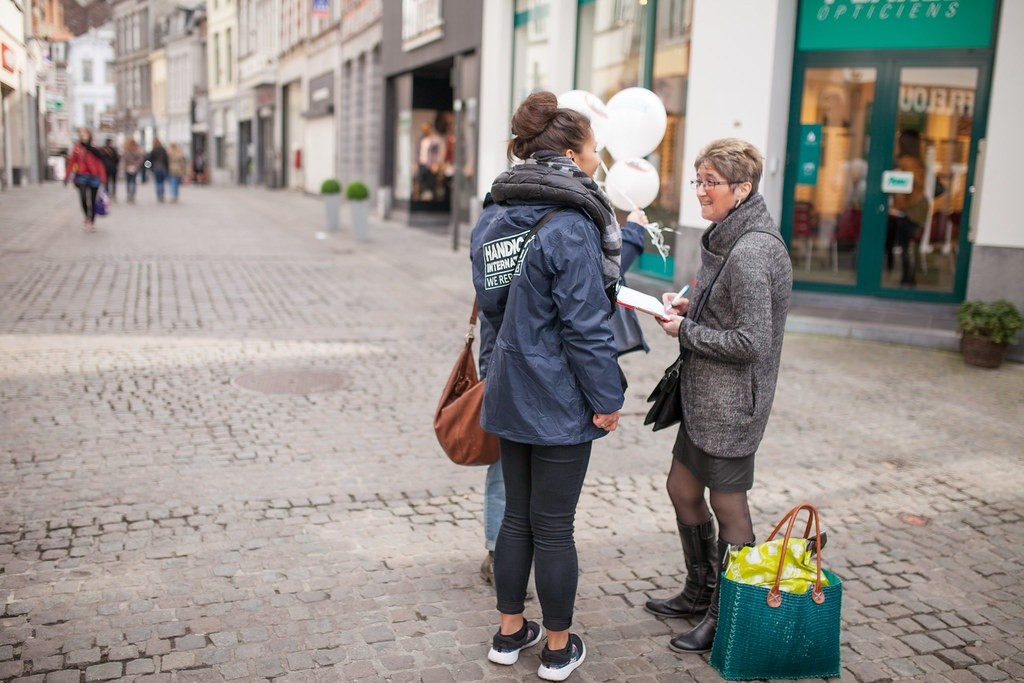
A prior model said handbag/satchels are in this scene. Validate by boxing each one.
[607,277,650,357]
[95,184,110,214]
[706,504,843,680]
[644,354,683,431]
[433,338,500,466]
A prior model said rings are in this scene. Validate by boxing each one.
[601,424,607,429]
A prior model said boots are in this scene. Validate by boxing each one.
[643,513,718,617]
[668,531,756,654]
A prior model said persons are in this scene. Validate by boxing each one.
[99,138,120,201]
[147,136,169,203]
[417,119,455,211]
[139,148,149,182]
[883,130,948,289]
[64,127,107,232]
[125,138,146,203]
[166,142,187,203]
[644,139,793,655]
[470,91,648,681]
[194,147,205,182]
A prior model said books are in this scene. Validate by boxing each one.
[615,285,670,322]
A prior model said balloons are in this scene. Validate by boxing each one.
[605,87,667,161]
[605,158,660,212]
[557,90,610,153]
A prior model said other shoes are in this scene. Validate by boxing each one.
[480,554,535,599]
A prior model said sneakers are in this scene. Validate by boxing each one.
[537,632,586,681]
[487,617,542,665]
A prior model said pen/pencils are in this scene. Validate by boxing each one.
[663,284,691,312]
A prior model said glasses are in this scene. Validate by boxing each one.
[691,179,744,190]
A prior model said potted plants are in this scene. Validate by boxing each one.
[306,179,340,228]
[957,300,1024,368]
[345,183,370,240]
